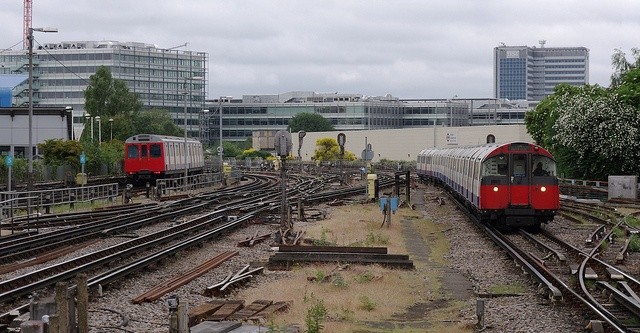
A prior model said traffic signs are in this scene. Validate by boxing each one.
[6,156,13,166]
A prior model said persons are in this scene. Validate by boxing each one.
[534,162,551,177]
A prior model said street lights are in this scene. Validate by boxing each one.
[85,114,93,154]
[65,106,73,140]
[184,77,202,184]
[28,27,58,182]
[219,96,233,171]
[95,116,101,156]
[199,110,209,142]
[109,118,114,140]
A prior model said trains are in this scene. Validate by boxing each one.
[123,134,204,186]
[417,142,559,225]
